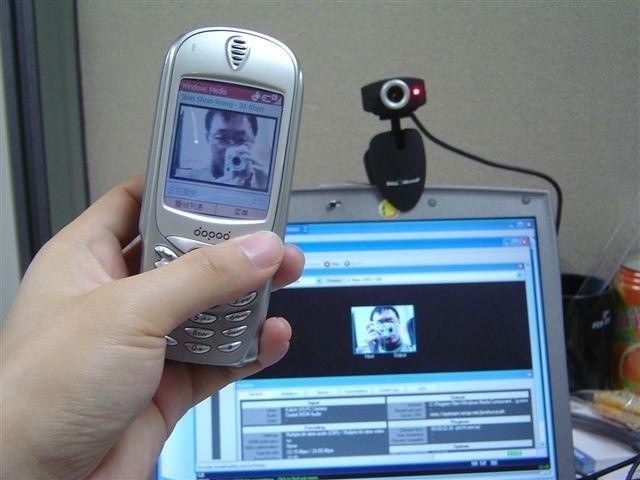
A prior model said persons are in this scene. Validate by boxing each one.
[0,174,307,480]
[356,307,411,352]
[189,109,269,192]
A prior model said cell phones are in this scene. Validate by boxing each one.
[136,28,303,369]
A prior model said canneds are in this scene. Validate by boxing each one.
[611,259,640,396]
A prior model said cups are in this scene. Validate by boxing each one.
[561,272,613,395]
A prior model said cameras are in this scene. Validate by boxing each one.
[222,146,250,187]
[375,323,395,335]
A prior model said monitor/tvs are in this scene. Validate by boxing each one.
[155,184,577,480]
